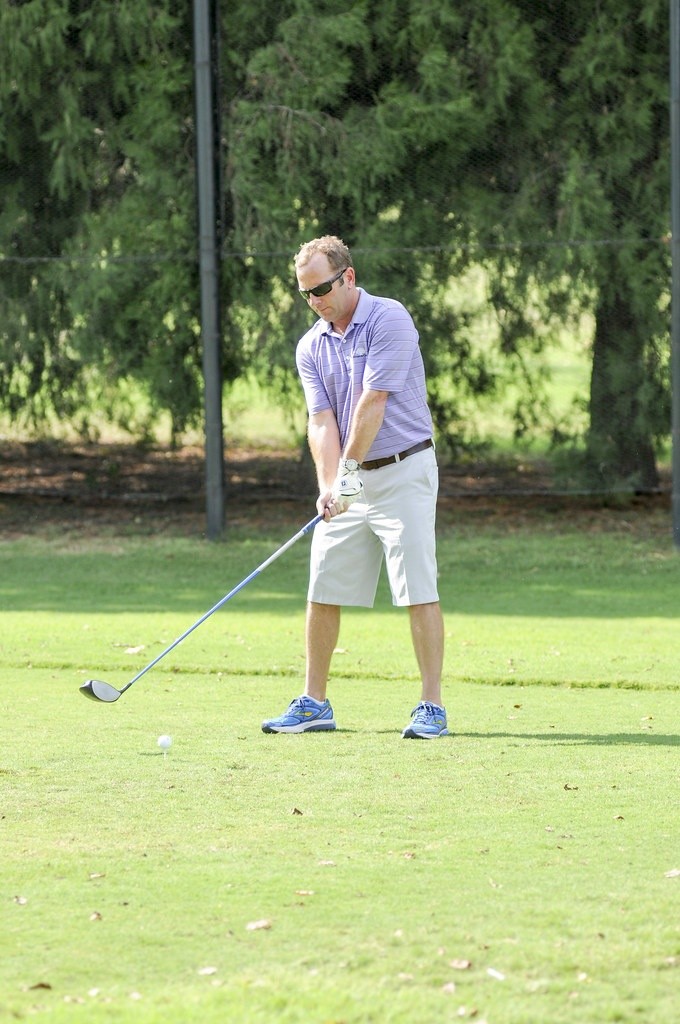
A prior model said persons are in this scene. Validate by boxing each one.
[261,235,448,739]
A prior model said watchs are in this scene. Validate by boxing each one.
[340,459,362,471]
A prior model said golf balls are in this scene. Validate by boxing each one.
[157,734,173,750]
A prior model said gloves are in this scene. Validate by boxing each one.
[331,458,363,512]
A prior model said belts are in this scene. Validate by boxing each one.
[360,438,433,470]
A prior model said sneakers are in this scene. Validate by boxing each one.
[402,701,448,739]
[261,696,336,734]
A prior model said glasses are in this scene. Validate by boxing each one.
[299,269,346,299]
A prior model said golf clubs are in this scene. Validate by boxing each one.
[78,511,326,704]
